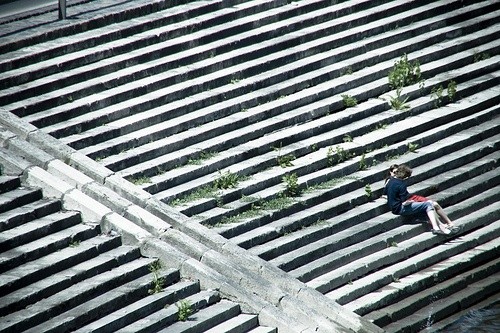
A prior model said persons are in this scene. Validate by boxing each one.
[382,164,460,234]
[387,165,451,235]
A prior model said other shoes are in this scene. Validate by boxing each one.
[432,229,444,234]
